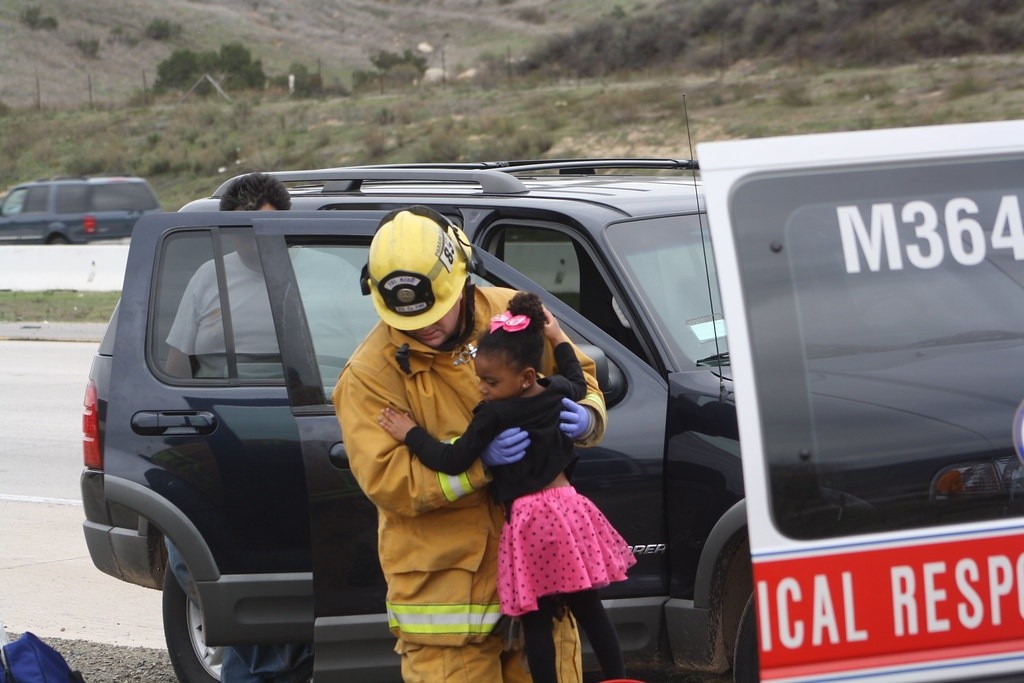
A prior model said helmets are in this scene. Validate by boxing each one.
[361,208,472,330]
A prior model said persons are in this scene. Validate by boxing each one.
[163,173,386,683]
[380,293,637,683]
[332,205,606,683]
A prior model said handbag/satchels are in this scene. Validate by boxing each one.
[0,630,84,683]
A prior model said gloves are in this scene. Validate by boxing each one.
[558,397,590,438]
[479,427,531,468]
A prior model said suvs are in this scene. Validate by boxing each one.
[0,176,160,245]
[77,158,1024,683]
